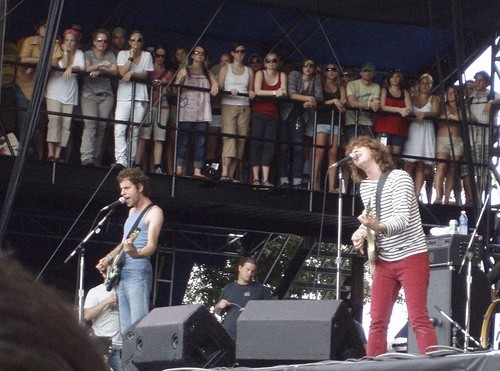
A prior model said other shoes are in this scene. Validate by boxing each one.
[82,159,95,165]
[252,180,275,187]
[192,174,208,179]
[47,156,64,163]
[220,176,241,183]
[279,184,303,190]
[464,202,473,207]
[434,199,442,204]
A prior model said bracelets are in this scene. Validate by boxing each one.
[273,90,276,96]
[366,101,370,108]
[128,57,134,62]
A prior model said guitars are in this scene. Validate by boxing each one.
[364,199,378,276]
[104,227,141,291]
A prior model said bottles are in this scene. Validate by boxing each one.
[459,211,468,235]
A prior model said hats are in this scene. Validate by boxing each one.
[419,70,433,81]
[361,62,375,72]
[64,29,80,40]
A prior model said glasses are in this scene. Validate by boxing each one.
[40,25,46,28]
[73,28,83,33]
[130,38,142,42]
[265,59,278,63]
[326,68,337,72]
[155,53,166,58]
[194,51,206,56]
[97,38,108,44]
[235,50,245,54]
[304,63,315,68]
[390,68,400,72]
[342,72,354,77]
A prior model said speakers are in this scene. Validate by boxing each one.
[236,299,367,367]
[121,314,146,371]
[134,303,236,371]
[406,265,491,355]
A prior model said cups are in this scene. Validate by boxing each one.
[449,220,458,234]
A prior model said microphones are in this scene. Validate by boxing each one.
[100,197,126,212]
[429,317,443,326]
[330,152,357,168]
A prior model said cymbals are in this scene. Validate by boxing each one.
[487,252,500,257]
[486,243,500,248]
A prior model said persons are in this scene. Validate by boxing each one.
[214,256,270,342]
[0,19,500,208]
[83,264,124,371]
[344,136,438,356]
[0,253,109,371]
[95,167,165,334]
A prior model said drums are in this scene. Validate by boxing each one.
[479,298,500,350]
